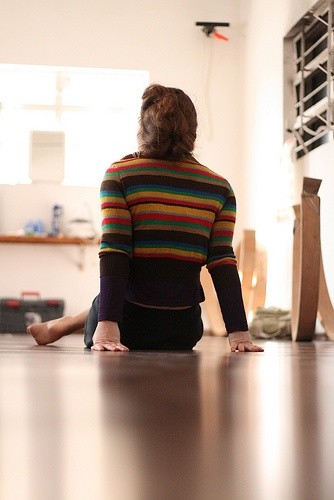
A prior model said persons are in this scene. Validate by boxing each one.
[26,84,263,353]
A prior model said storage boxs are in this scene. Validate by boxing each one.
[0,291,65,335]
